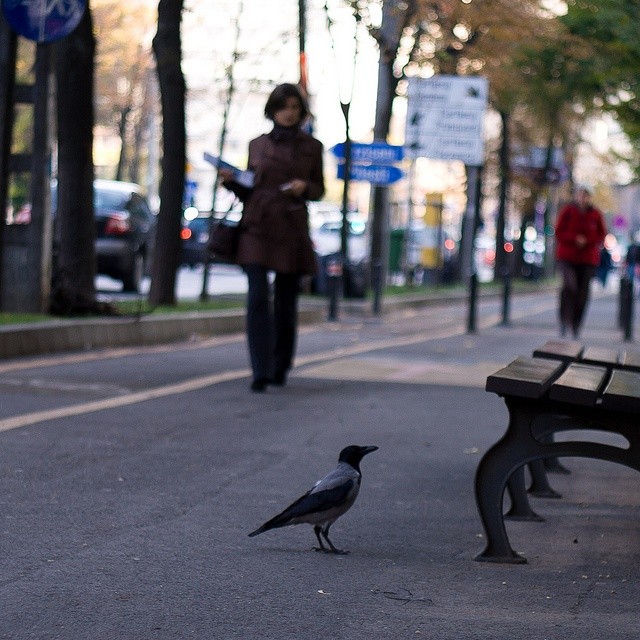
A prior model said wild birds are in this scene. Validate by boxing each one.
[248,445,380,555]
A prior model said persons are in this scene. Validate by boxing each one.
[204,84,327,394]
[550,184,607,343]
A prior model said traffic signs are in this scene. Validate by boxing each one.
[337,163,403,186]
[328,141,404,165]
[406,78,487,166]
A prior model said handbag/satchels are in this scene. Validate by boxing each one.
[207,192,246,257]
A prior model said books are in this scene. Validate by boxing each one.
[205,153,255,190]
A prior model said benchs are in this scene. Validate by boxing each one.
[470,336,638,564]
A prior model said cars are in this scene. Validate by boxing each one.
[48,178,157,295]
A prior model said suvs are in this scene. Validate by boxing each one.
[310,216,370,299]
[182,216,247,274]
